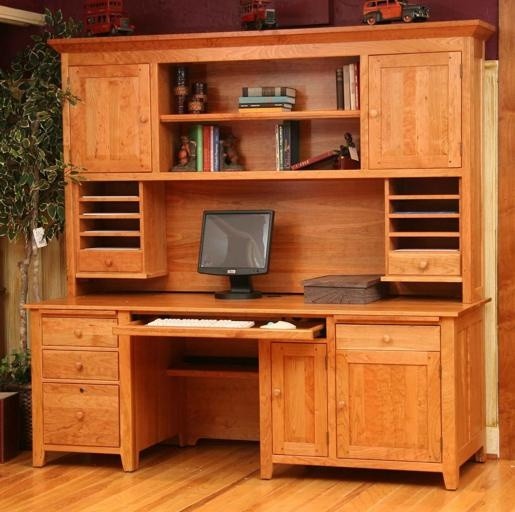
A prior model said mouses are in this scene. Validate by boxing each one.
[260,319,297,330]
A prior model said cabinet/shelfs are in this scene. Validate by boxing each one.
[42,35,483,306]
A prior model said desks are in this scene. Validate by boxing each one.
[20,293,490,488]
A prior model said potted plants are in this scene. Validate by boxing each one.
[0,6,85,452]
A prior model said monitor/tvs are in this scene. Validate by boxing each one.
[196,209,276,300]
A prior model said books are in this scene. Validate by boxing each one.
[336,62,360,111]
[274,119,341,171]
[238,86,296,114]
[196,124,220,171]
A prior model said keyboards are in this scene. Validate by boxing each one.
[147,317,255,329]
